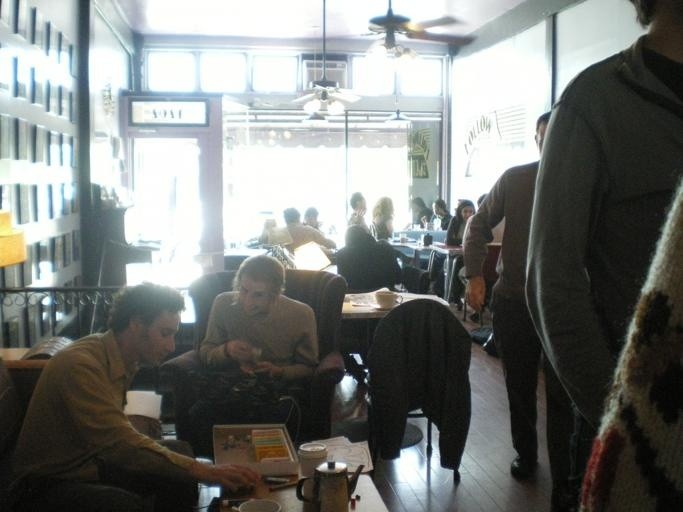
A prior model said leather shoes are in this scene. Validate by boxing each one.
[511,455,538,479]
[483,333,497,355]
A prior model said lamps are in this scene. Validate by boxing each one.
[301,96,347,117]
[384,67,413,128]
[367,39,420,61]
[0,211,27,289]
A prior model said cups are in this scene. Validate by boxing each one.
[296,441,328,477]
[234,497,281,512]
[374,289,405,308]
[398,232,408,247]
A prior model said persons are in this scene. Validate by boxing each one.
[0,280,259,511]
[251,188,506,357]
[524,0,683,512]
[174,255,320,456]
[462,109,574,481]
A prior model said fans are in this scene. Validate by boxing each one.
[292,1,360,118]
[360,0,475,62]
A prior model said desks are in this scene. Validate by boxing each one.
[197,435,391,512]
[180,293,448,329]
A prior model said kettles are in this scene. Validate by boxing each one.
[295,457,366,511]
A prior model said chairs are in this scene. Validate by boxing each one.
[0,357,196,511]
[347,230,500,485]
[187,267,349,444]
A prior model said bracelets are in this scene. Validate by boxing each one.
[223,341,232,360]
